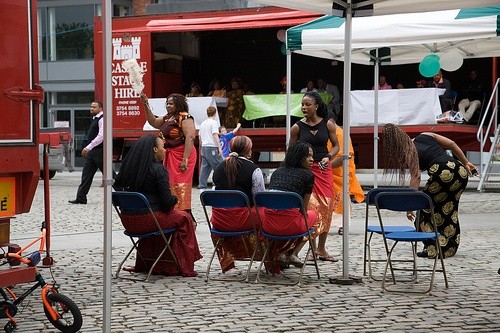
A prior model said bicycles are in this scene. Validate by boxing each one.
[0,220,83,333]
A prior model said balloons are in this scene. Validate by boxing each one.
[419,49,463,79]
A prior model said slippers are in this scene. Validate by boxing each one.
[319,255,338,262]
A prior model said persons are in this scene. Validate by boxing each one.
[395,82,404,89]
[300,81,318,94]
[317,77,342,122]
[267,141,321,268]
[69,102,118,204]
[426,71,452,114]
[370,74,394,90]
[187,83,202,98]
[381,123,480,259]
[416,79,427,87]
[223,78,245,128]
[198,108,242,188]
[278,74,293,94]
[287,92,365,261]
[207,81,227,98]
[213,136,266,274]
[139,92,197,229]
[112,134,202,278]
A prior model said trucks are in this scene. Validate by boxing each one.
[0,1,71,262]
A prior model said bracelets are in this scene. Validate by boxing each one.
[182,158,189,161]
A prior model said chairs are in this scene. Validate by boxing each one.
[362,187,420,282]
[111,191,181,283]
[200,190,271,283]
[253,192,321,287]
[451,90,486,126]
[375,191,449,297]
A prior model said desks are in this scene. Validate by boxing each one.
[143,96,228,132]
[349,88,447,127]
[241,91,334,128]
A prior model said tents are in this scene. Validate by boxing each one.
[246,0,500,284]
[286,5,500,161]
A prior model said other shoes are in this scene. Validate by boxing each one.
[68,199,87,204]
[290,256,304,267]
